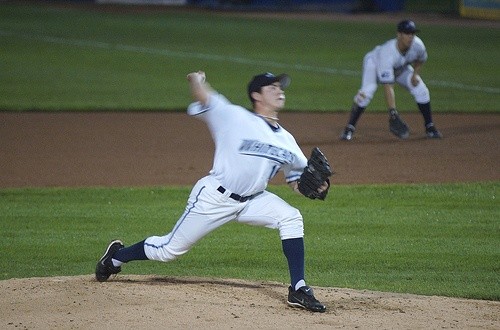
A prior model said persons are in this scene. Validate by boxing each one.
[96,70,331,311]
[339,20,444,140]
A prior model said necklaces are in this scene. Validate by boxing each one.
[256,113,279,121]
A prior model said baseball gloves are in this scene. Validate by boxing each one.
[388,108,409,141]
[298,147,335,201]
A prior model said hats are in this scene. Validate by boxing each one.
[248,73,291,101]
[398,20,421,34]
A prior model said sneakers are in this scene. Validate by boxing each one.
[96,239,124,282]
[425,126,444,139]
[288,284,326,313]
[341,128,353,143]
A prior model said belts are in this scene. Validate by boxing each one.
[217,186,264,202]
